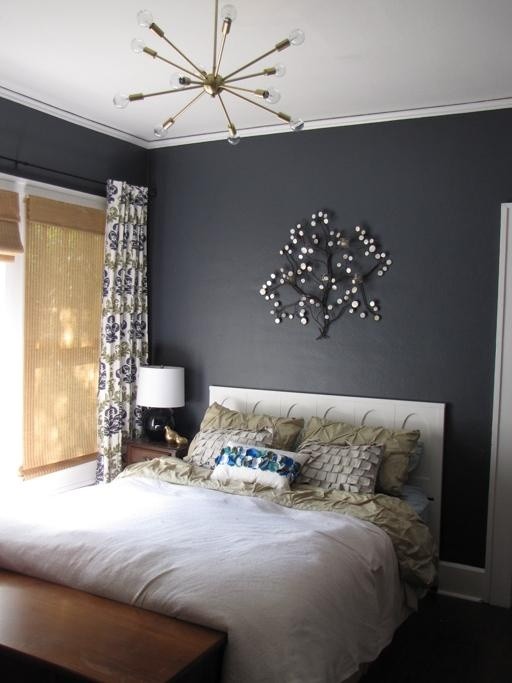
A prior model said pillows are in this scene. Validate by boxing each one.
[183,401,425,495]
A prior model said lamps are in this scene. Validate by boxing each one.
[112,1,306,145]
[135,363,187,442]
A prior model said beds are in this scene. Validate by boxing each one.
[0,384,446,682]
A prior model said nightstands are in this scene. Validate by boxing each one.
[126,439,189,467]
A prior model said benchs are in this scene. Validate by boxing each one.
[0,570,229,680]
